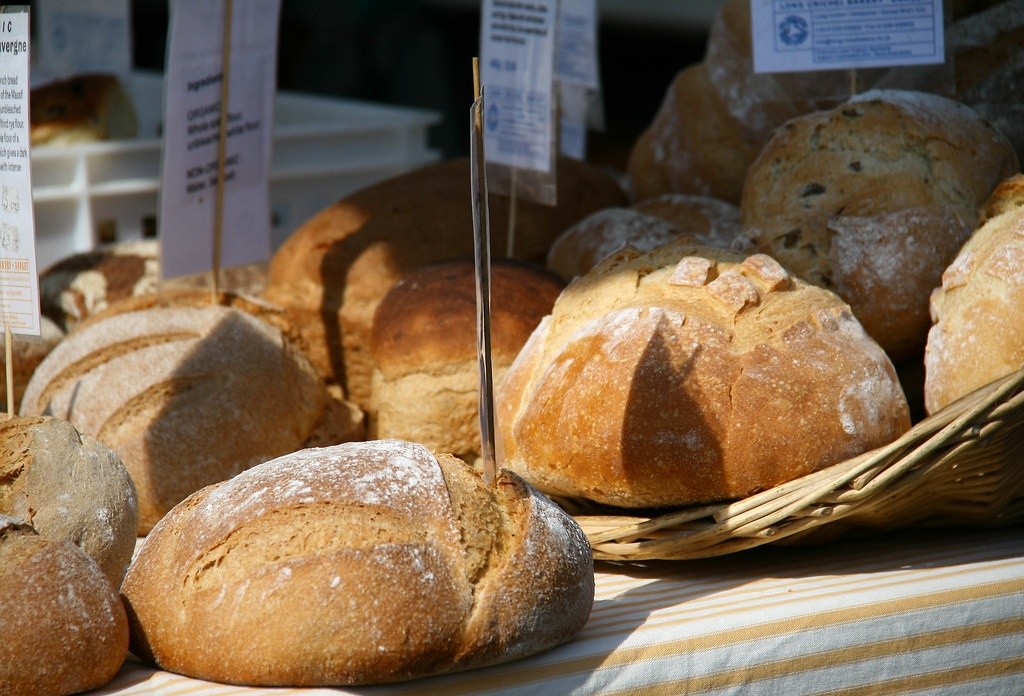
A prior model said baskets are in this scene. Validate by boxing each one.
[461,365,1024,564]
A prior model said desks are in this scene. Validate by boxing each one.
[83,516,1024,696]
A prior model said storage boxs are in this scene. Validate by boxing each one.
[29,77,433,274]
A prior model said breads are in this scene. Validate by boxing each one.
[0,0,1024,696]
